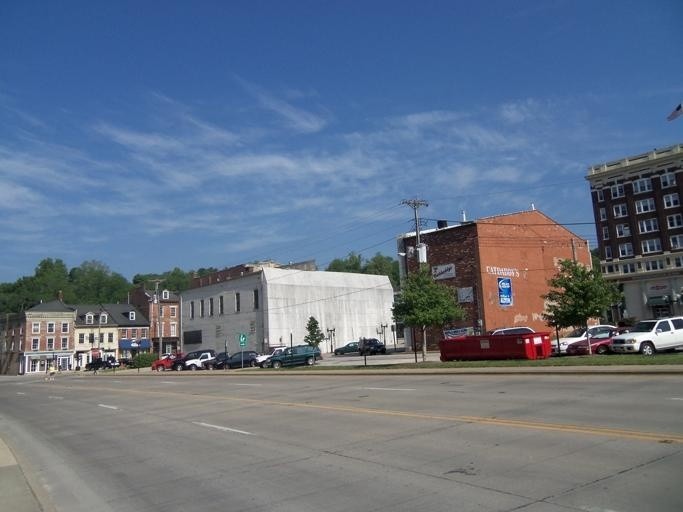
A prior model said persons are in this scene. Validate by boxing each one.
[49,362,55,384]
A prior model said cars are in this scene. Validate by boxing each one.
[564,326,634,356]
[85,355,121,371]
[608,315,682,357]
[265,345,323,370]
[152,350,259,370]
[118,357,134,366]
[358,338,387,357]
[255,346,288,368]
[550,324,618,357]
[334,341,361,355]
[490,327,536,336]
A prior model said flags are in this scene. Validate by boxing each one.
[667,103,683,122]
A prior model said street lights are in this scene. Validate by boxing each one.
[662,288,681,316]
[396,236,417,362]
[144,279,163,359]
